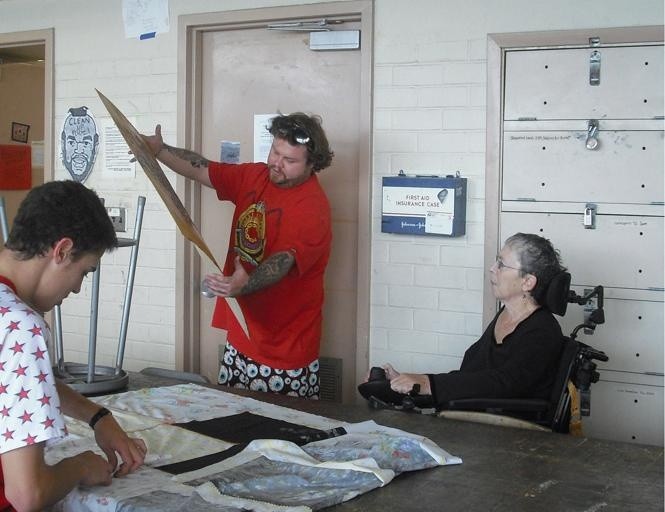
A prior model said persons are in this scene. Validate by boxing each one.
[60,106,101,185]
[362,231,565,432]
[0,179,148,510]
[127,111,336,400]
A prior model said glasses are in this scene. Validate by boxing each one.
[495,251,527,271]
[270,115,312,144]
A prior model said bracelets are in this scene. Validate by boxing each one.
[88,406,113,430]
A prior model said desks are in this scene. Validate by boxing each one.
[53,362,665,510]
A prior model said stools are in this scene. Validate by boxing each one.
[0,193,143,394]
